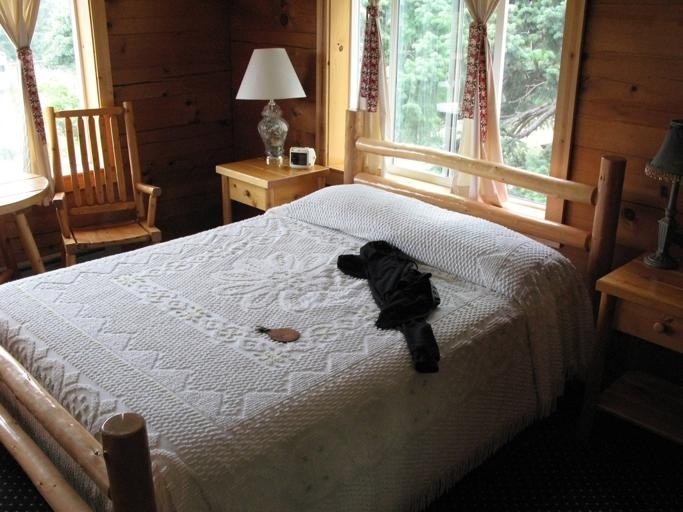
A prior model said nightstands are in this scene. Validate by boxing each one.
[214,158,332,225]
[579,246,682,447]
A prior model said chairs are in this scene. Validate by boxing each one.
[45,99,162,269]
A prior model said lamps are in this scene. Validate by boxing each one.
[233,46,307,168]
[636,117,682,270]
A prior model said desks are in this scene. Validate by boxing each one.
[0,170,49,285]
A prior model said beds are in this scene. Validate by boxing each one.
[0,109,626,512]
